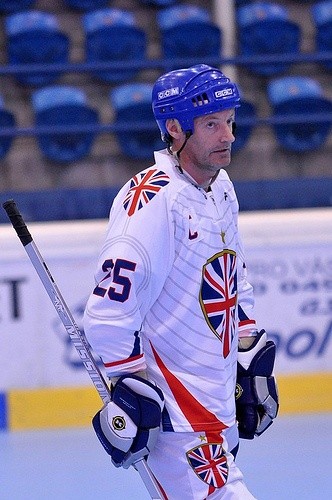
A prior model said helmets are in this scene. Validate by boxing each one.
[152,64,240,140]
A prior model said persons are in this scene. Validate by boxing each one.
[82,63,279,500]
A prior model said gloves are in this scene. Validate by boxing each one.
[236,329,279,440]
[91,374,166,468]
